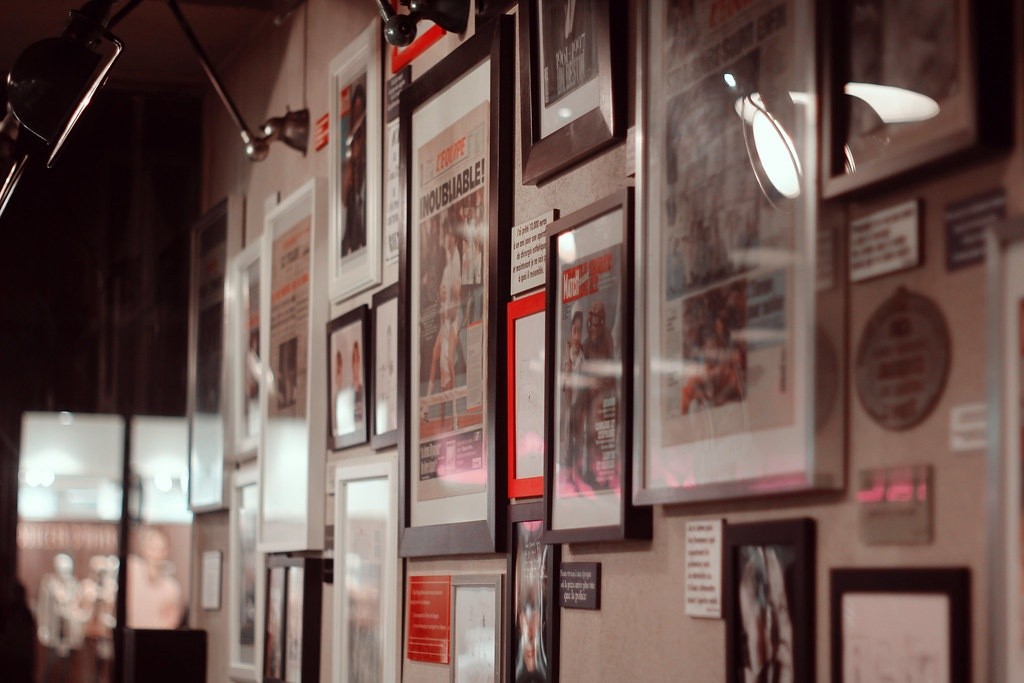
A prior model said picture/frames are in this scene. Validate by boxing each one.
[185,0,1024,683]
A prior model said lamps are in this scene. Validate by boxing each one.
[0,0,472,218]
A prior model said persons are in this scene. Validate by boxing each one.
[666,60,758,417]
[37,531,184,683]
[333,342,363,436]
[516,593,547,683]
[562,302,620,493]
[416,191,484,434]
[735,544,797,683]
